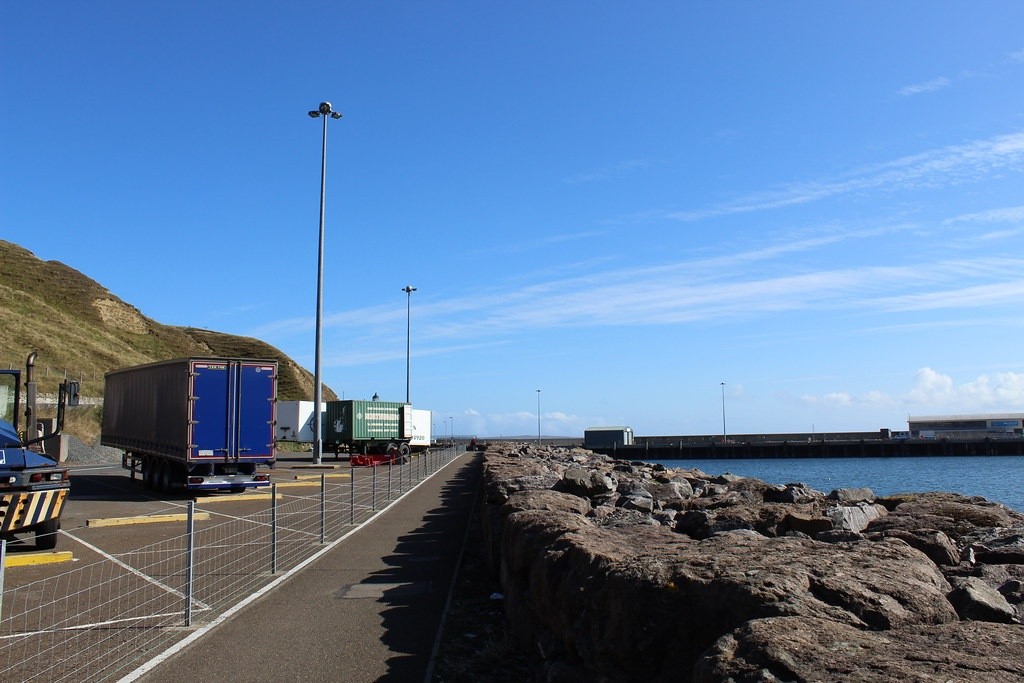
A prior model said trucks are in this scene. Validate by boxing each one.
[0,351,83,548]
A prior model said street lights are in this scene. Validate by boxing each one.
[304,99,345,465]
[449,416,453,437]
[720,381,728,444]
[401,286,418,402]
[536,388,543,445]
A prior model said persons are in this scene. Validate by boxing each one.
[471,438,476,451]
